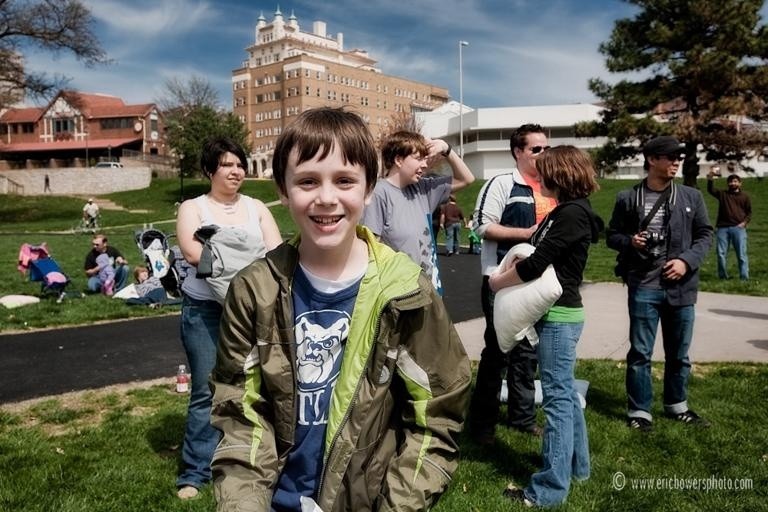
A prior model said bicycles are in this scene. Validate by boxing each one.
[71,213,104,235]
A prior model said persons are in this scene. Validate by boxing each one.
[360,131,475,300]
[83,199,99,229]
[432,194,481,257]
[707,169,752,279]
[466,214,482,252]
[177,133,283,503]
[489,145,605,507]
[605,136,716,431]
[112,265,167,311]
[44,174,51,193]
[207,108,472,512]
[461,124,558,458]
[84,234,130,295]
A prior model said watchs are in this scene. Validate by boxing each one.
[441,142,452,156]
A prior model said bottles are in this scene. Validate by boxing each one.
[177,365,189,393]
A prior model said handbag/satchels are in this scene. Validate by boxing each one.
[614,186,671,280]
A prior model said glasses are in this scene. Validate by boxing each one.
[92,243,99,247]
[660,154,683,161]
[526,145,551,153]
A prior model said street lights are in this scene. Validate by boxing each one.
[459,40,470,160]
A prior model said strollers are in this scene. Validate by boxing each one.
[17,241,86,304]
[134,228,170,278]
[163,232,193,298]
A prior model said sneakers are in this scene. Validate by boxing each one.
[526,424,545,435]
[630,417,652,432]
[677,412,711,429]
[447,250,453,257]
[503,488,527,505]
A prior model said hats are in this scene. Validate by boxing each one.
[88,199,93,202]
[642,136,686,154]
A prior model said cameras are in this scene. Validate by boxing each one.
[641,231,666,253]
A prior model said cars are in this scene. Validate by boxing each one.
[96,161,124,168]
